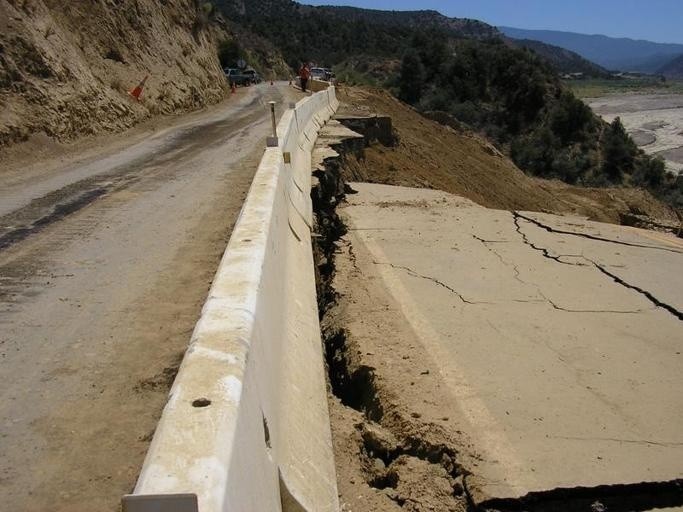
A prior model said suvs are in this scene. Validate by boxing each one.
[310,68,331,77]
[223,68,250,87]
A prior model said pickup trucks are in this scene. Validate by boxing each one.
[243,69,261,85]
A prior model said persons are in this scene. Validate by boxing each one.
[299,62,312,93]
